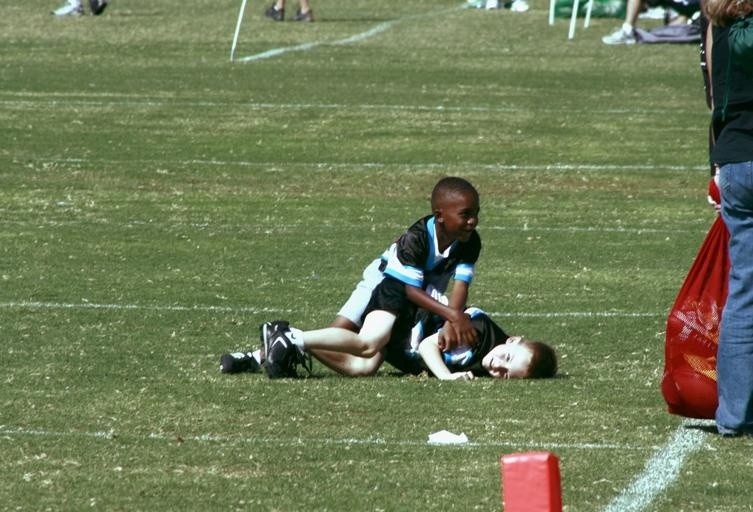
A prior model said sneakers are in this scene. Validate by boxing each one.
[260,319,297,379]
[50,2,86,17]
[510,0,530,11]
[486,0,500,9]
[219,351,256,374]
[287,9,315,23]
[264,3,284,21]
[602,22,636,45]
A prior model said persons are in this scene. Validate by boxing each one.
[601,0,702,45]
[49,0,113,18]
[261,0,319,25]
[214,175,482,375]
[255,272,560,384]
[700,0,752,448]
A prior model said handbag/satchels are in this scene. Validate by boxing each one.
[661,197,730,420]
